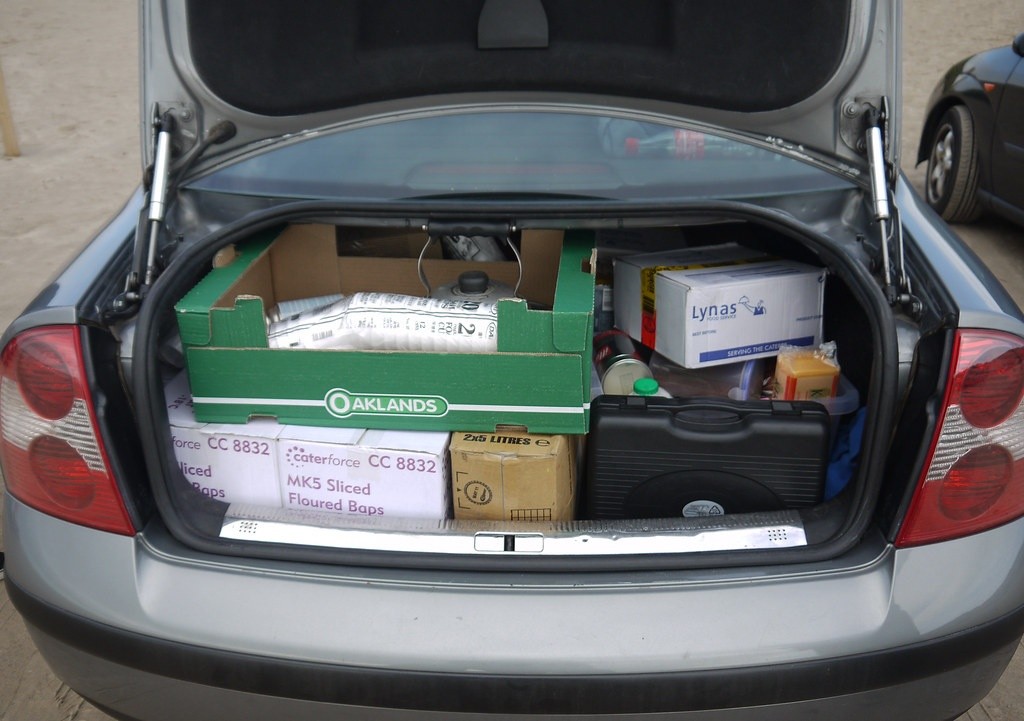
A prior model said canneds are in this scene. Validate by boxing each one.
[591,329,654,395]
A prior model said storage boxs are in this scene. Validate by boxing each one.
[164,364,288,509]
[173,223,601,435]
[609,244,829,370]
[448,430,588,521]
[579,394,832,520]
[275,423,453,520]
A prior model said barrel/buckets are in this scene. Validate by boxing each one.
[417,217,524,304]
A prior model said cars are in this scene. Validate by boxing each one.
[911,31,1024,229]
[0,0,1024,721]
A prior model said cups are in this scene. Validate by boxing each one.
[649,349,765,403]
[594,327,654,396]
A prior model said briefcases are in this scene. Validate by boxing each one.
[583,393,831,518]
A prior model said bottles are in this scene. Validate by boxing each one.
[628,378,673,398]
[266,294,344,323]
